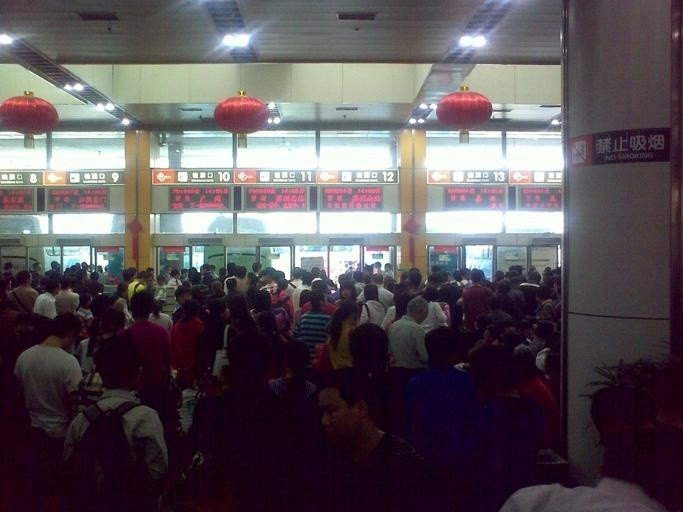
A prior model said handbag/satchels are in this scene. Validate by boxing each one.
[212,325,232,377]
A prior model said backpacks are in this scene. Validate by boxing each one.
[61,399,149,512]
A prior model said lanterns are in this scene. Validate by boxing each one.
[212,90,271,150]
[0,90,59,149]
[434,84,493,144]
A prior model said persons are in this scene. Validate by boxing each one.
[499,359,681,509]
[0,260,560,510]
